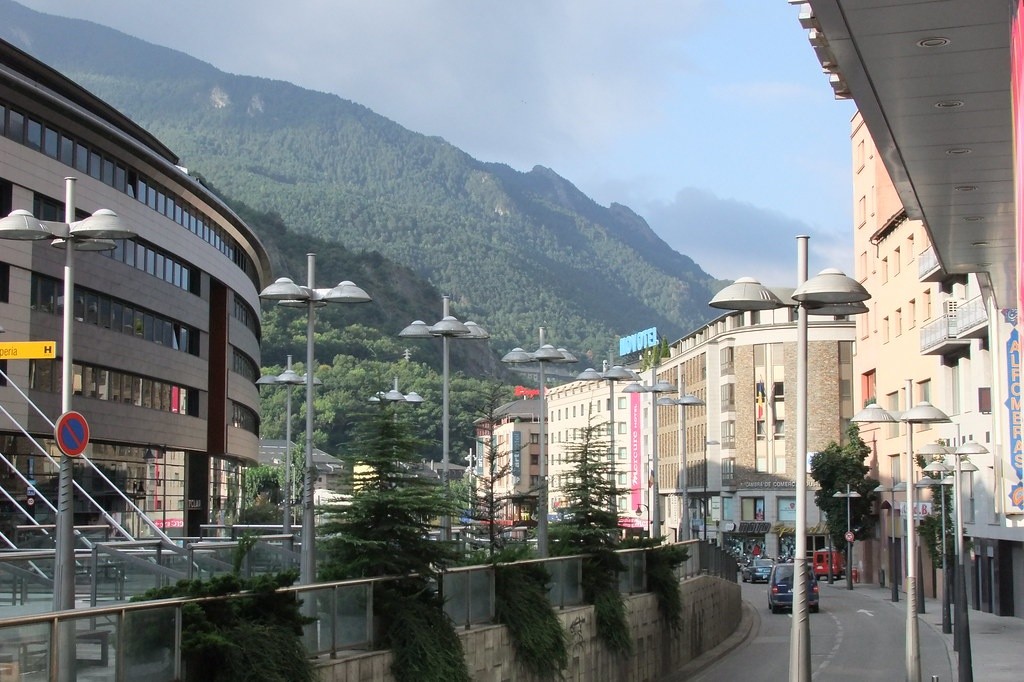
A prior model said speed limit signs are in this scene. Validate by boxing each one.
[27,498,34,505]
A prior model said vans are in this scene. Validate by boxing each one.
[768,563,819,614]
[813,551,842,580]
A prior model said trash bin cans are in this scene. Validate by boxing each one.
[852,569,857,583]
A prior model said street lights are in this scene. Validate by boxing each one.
[259,252,372,660]
[849,378,953,682]
[369,377,425,462]
[621,366,679,545]
[688,435,719,540]
[916,425,990,682]
[635,453,660,538]
[654,373,705,541]
[832,484,862,590]
[709,235,871,682]
[0,178,139,682]
[256,354,324,575]
[500,326,579,560]
[576,350,642,543]
[396,294,490,541]
[873,477,915,602]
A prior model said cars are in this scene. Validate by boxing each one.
[741,559,775,584]
